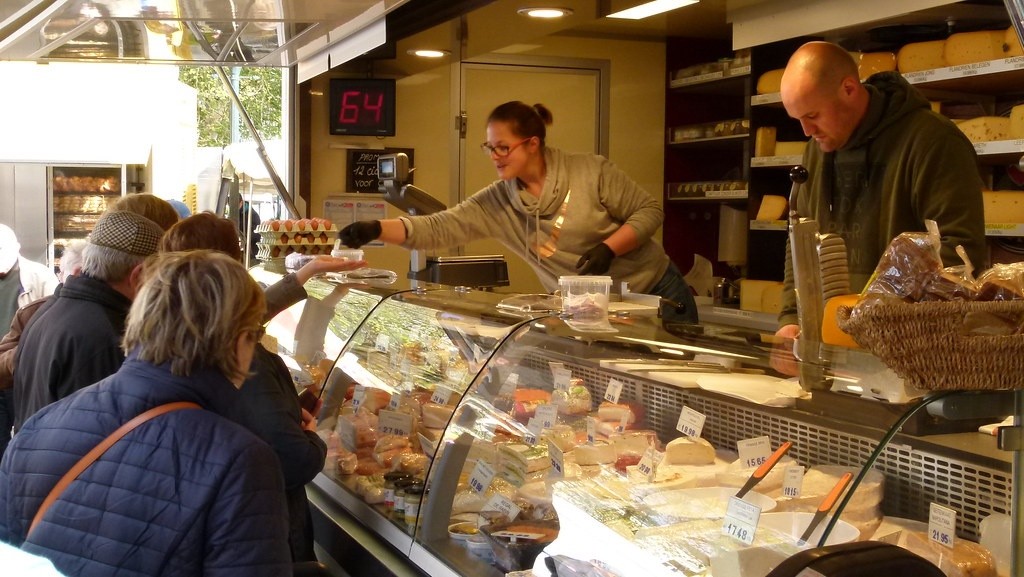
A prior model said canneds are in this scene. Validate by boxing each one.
[383,472,432,528]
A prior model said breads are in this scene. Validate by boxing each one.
[853,237,1024,310]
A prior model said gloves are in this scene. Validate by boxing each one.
[576,243,616,275]
[337,220,382,249]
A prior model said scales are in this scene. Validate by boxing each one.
[378,152,509,292]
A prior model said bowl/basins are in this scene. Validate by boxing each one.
[332,248,364,263]
[556,274,613,321]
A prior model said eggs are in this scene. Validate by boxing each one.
[261,220,332,257]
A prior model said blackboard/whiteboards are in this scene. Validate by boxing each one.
[347,147,413,194]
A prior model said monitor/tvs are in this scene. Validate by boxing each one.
[329,78,396,137]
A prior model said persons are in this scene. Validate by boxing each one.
[0,192,369,577]
[338,101,699,334]
[228,192,261,259]
[769,41,987,379]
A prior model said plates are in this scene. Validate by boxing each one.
[743,510,859,549]
[645,486,776,519]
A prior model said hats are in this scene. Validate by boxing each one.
[90,211,164,257]
[167,198,190,218]
[0,223,20,275]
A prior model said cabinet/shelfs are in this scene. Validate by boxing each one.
[0,145,153,282]
[664,64,750,199]
[749,55,1023,237]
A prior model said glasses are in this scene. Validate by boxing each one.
[480,138,530,157]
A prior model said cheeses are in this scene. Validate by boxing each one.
[753,24,1024,158]
[821,294,864,347]
[756,193,789,220]
[739,280,786,315]
[422,401,720,486]
[981,190,1024,226]
